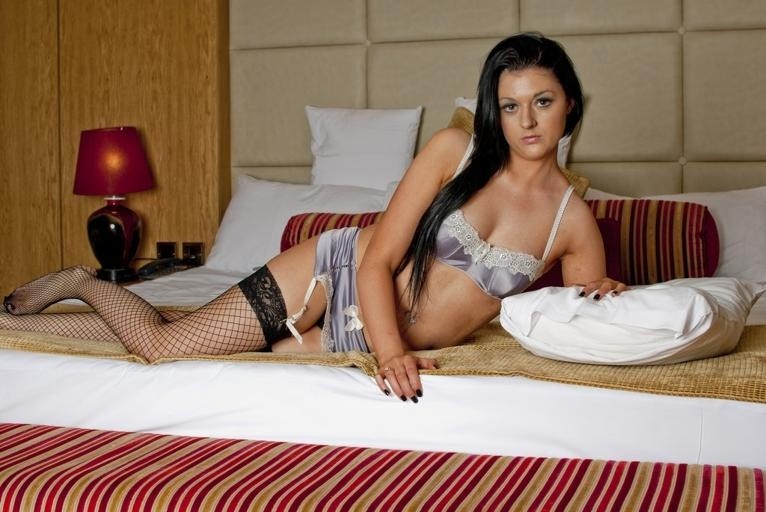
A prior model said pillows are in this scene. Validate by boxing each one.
[583,184,765,285]
[453,98,570,167]
[305,104,428,191]
[277,197,719,285]
[204,174,388,274]
[499,275,766,368]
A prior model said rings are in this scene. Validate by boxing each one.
[383,366,393,374]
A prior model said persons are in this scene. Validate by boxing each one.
[1,30,632,404]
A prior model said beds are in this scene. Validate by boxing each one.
[0,258,765,510]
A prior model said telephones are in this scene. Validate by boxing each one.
[137,256,196,279]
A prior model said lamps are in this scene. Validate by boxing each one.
[74,123,163,288]
